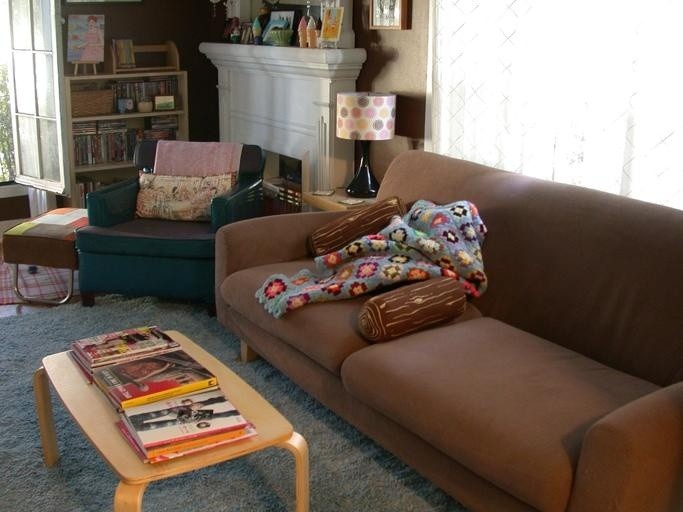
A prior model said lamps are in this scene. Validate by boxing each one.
[336,91,399,197]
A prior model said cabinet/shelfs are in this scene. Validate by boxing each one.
[63,70,190,208]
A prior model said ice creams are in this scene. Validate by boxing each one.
[297,15,307,48]
[252,15,262,45]
[305,16,316,48]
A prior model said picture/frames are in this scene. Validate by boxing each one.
[368,0,408,31]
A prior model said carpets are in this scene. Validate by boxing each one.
[0,294,468,511]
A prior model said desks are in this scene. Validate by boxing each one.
[302,189,376,211]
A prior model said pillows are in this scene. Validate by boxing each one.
[309,194,406,257]
[134,169,237,226]
[358,273,472,343]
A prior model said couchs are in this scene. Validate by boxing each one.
[213,147,682,511]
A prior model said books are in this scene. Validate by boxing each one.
[68,325,256,465]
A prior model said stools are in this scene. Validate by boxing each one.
[2,206,89,306]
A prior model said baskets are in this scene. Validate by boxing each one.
[270,30,294,46]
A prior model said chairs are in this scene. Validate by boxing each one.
[75,139,266,317]
